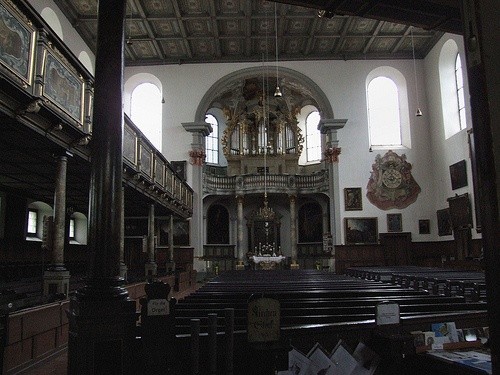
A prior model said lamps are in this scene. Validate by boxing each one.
[410,25,423,116]
[273,2,283,97]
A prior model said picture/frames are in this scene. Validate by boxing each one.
[386,213,402,232]
[449,159,467,190]
[343,216,379,244]
[419,219,430,234]
[344,187,362,211]
